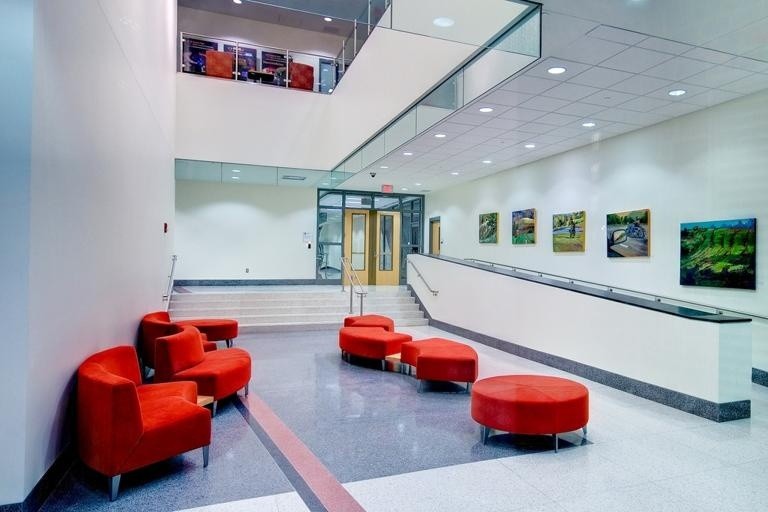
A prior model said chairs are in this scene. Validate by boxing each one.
[76,309,256,500]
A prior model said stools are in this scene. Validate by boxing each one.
[343,309,592,450]
